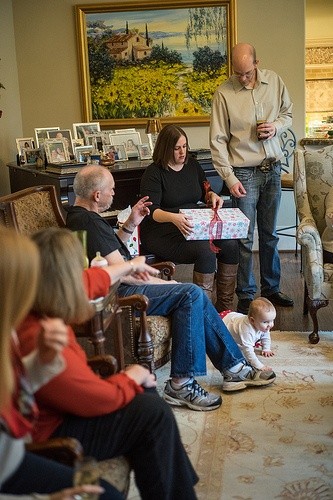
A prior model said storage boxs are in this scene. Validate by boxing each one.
[179,208,250,241]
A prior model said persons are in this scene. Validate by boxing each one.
[22,126,148,162]
[66,164,276,411]
[0,224,105,500]
[135,125,239,313]
[209,43,294,315]
[18,227,199,500]
[219,297,276,371]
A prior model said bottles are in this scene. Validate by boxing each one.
[91,251,108,268]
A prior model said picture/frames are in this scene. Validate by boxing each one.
[15,0,240,164]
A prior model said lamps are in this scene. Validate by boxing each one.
[144,120,162,145]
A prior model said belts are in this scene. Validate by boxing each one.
[237,160,281,173]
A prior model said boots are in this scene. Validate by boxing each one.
[193,270,215,306]
[215,260,239,313]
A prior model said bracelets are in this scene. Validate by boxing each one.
[122,226,133,234]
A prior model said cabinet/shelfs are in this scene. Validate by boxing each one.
[8,150,232,226]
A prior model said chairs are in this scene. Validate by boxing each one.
[294,139,333,345]
[0,187,177,500]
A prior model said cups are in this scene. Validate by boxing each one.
[257,120,269,141]
[73,456,99,500]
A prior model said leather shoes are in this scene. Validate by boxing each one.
[261,292,294,307]
[237,300,252,315]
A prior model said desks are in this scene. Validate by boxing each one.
[70,280,127,380]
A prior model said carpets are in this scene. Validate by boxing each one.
[126,330,333,500]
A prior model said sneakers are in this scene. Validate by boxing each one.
[163,376,222,411]
[221,361,276,392]
